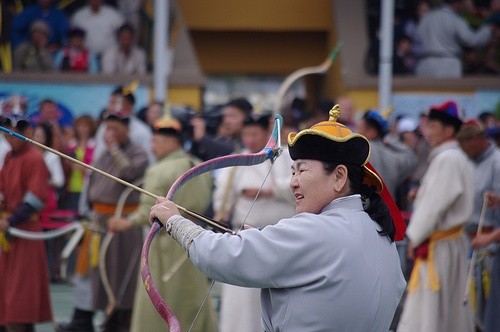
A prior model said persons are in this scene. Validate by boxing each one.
[148,104,407,332]
[0,84,329,332]
[361,100,500,332]
[368,0,500,80]
[10,0,153,76]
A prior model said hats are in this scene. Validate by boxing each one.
[106,104,129,127]
[428,101,463,132]
[114,79,140,104]
[150,99,181,136]
[287,104,383,192]
[362,104,394,137]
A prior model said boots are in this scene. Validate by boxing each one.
[55,306,95,332]
[100,307,133,332]
